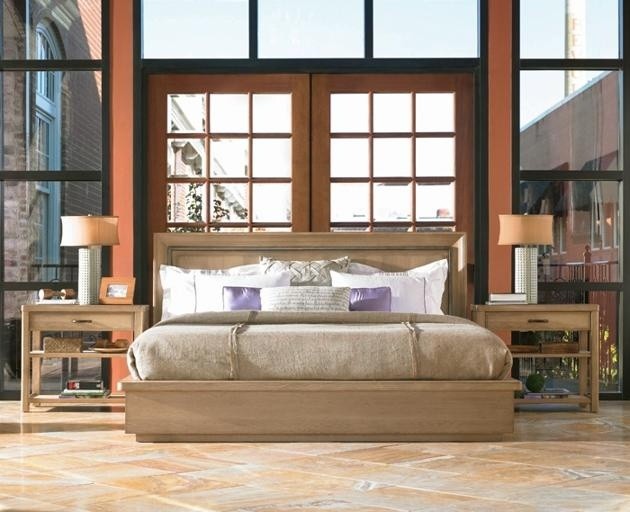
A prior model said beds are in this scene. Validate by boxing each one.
[117,231,523,442]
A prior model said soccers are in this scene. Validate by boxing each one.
[526,372,546,392]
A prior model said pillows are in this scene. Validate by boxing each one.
[157,255,451,317]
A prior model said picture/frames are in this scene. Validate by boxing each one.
[99,276,137,304]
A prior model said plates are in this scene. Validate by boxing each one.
[93,346,129,353]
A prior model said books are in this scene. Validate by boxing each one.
[58,380,125,399]
[524,388,568,399]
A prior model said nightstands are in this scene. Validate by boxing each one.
[470,303,600,413]
[20,303,150,413]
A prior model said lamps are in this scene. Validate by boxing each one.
[496,212,555,304]
[59,213,120,306]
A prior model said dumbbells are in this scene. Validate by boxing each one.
[96,338,128,348]
[39,289,75,300]
